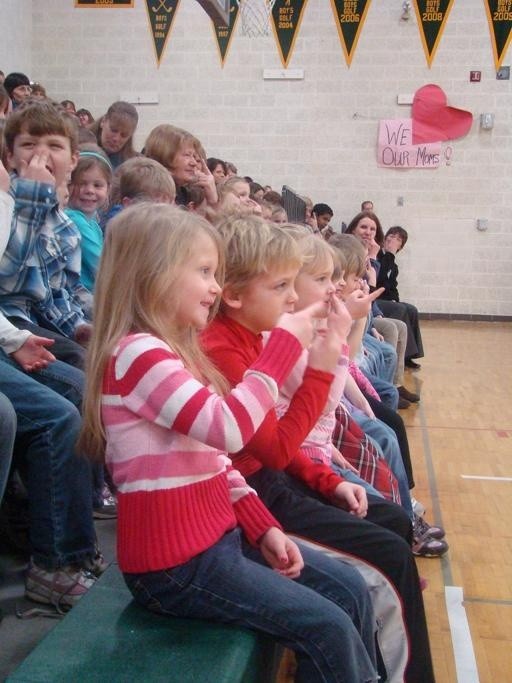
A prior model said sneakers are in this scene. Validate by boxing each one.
[411,494,448,557]
[25,546,109,608]
[397,385,420,409]
[406,359,420,369]
[93,484,119,519]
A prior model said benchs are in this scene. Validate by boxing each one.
[1,539,286,680]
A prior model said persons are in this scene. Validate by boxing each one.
[76,202,448,683]
[0,73,336,620]
